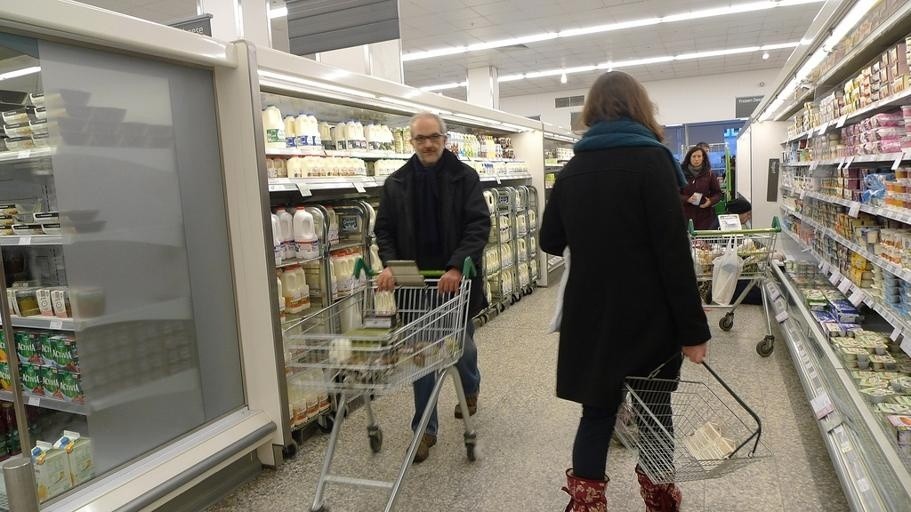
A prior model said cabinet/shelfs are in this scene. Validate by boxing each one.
[1,39,200,474]
[770,6,911,512]
[256,70,583,439]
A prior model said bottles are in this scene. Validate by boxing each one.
[395,127,515,158]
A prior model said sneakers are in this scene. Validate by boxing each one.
[405,429,440,465]
[454,384,481,419]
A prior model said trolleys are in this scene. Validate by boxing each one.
[279,253,481,512]
[683,215,782,358]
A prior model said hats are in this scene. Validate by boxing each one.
[725,191,752,214]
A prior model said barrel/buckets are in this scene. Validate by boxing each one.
[29,430,94,504]
[262,104,539,426]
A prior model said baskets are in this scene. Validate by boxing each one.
[611,340,780,490]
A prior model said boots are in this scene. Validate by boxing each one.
[560,465,613,512]
[633,460,686,512]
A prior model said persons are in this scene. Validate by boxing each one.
[676,147,722,232]
[695,142,710,155]
[535,71,712,512]
[710,198,763,305]
[720,155,735,198]
[373,109,493,463]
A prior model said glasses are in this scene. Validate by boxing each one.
[411,131,446,143]
